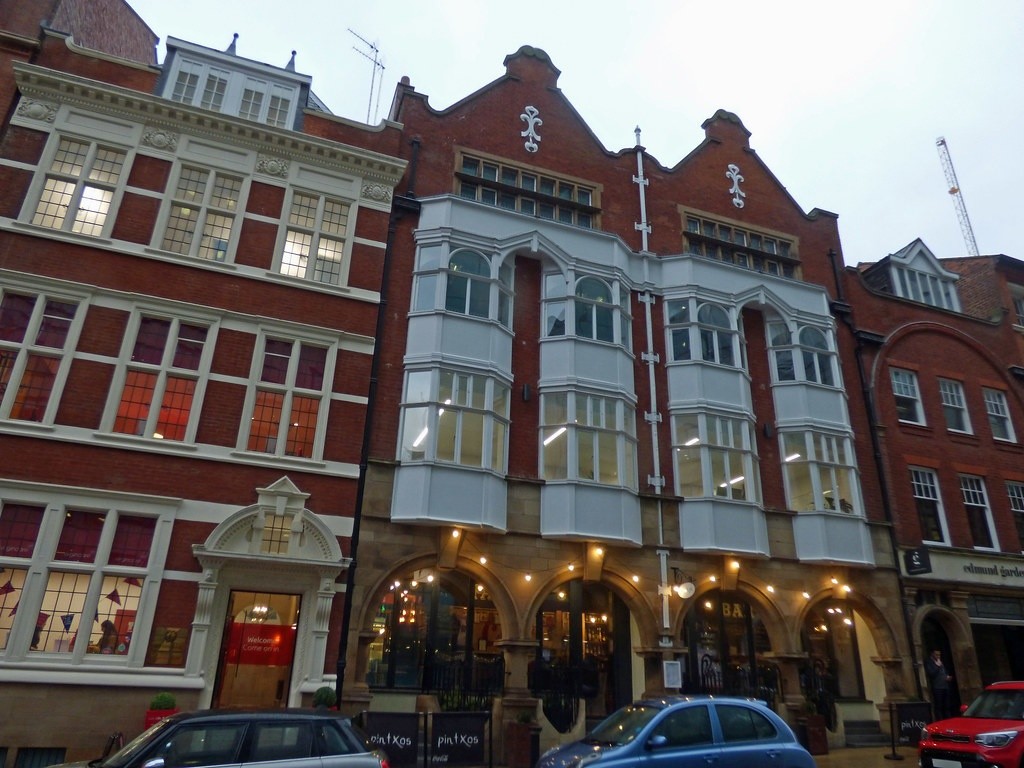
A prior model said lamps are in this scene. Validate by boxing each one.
[251,594,276,624]
[673,566,696,599]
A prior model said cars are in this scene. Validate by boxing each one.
[45,708,392,768]
[536,694,817,768]
[918,681,1024,768]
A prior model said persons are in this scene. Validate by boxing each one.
[95,619,117,654]
[926,649,953,718]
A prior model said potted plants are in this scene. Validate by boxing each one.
[311,686,338,712]
[143,691,179,732]
[797,700,830,757]
